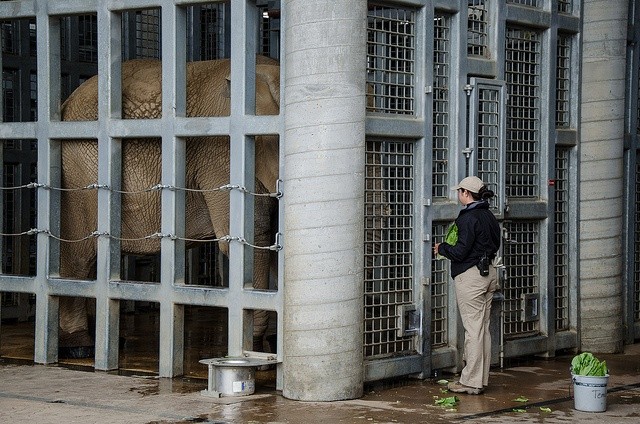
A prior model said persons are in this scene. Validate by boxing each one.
[436,176,500,395]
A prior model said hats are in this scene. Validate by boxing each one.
[450,175,484,194]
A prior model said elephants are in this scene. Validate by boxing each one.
[60,54,391,370]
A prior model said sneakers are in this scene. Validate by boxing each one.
[447,381,484,394]
